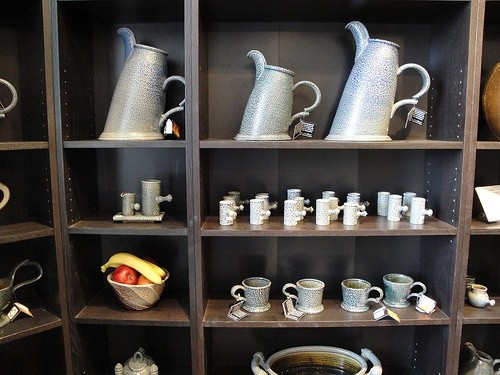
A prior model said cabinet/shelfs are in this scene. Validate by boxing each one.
[0,0,500,375]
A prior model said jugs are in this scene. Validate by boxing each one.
[94,26,185,140]
[323,21,431,140]
[460,341,500,375]
[231,47,320,140]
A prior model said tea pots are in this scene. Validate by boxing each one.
[0,256,43,326]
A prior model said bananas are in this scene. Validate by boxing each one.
[100,253,166,285]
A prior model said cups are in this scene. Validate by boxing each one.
[120,181,173,214]
[465,276,495,309]
[217,190,435,226]
[281,278,325,314]
[341,278,384,312]
[382,274,426,308]
[232,276,273,313]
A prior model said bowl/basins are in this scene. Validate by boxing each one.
[106,267,169,311]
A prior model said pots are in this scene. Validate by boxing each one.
[252,344,382,375]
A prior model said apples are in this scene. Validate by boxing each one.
[110,266,155,285]
